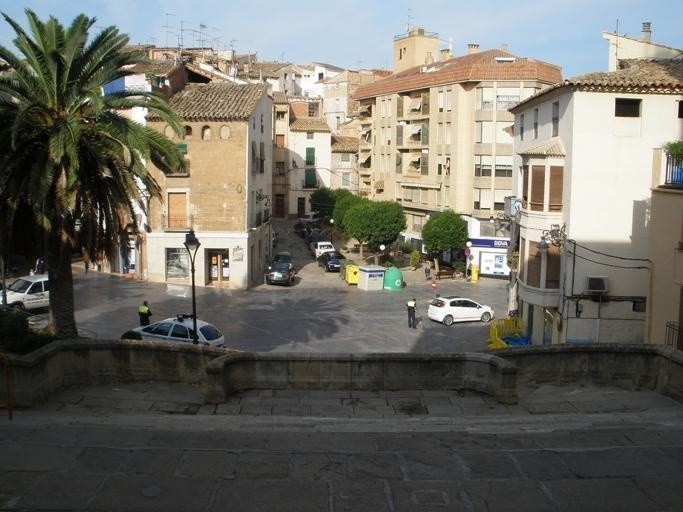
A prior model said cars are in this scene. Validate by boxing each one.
[427,296,495,326]
[265,252,296,286]
[0,275,50,312]
[300,212,324,222]
[131,313,227,348]
[294,221,346,272]
[272,231,278,248]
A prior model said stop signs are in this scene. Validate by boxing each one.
[431,281,438,290]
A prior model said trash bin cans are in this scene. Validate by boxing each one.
[470,265,479,281]
[345,264,359,285]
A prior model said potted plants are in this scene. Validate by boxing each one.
[663,140,682,186]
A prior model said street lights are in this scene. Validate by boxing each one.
[330,219,335,244]
[182,229,201,344]
[465,240,473,280]
[379,245,385,266]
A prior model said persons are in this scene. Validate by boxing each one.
[138,301,152,326]
[30,255,45,275]
[406,298,418,329]
[425,267,430,279]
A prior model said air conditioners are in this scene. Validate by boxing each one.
[586,277,608,294]
